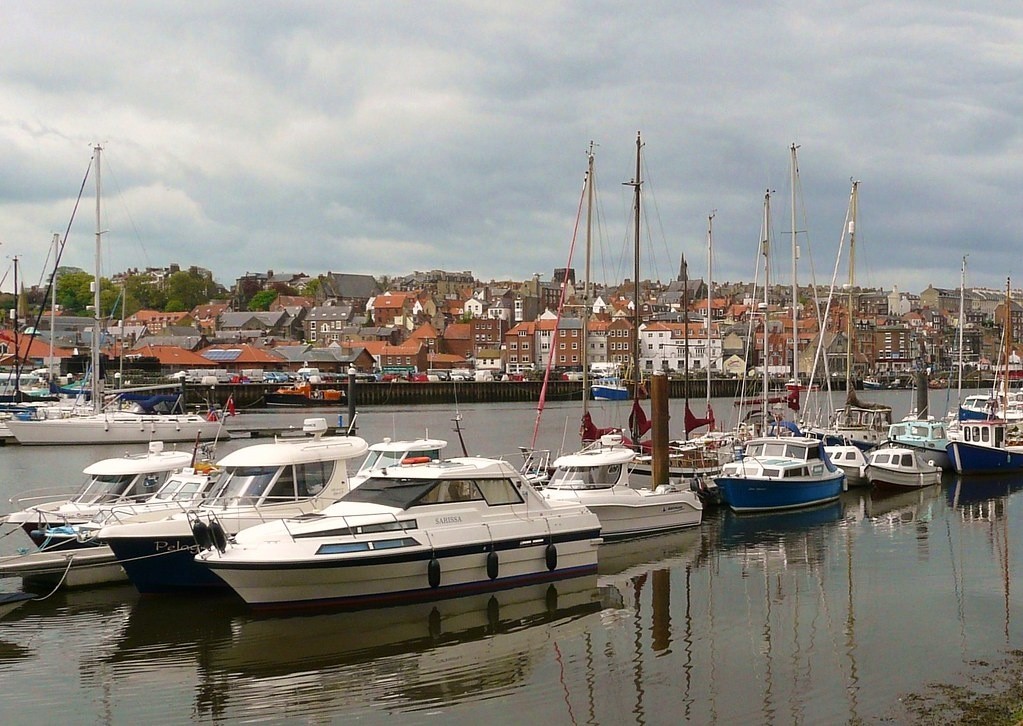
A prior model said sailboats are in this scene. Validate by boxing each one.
[1,128,1023,515]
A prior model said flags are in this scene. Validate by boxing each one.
[229,398,235,417]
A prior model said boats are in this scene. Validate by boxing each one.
[0,425,708,607]
